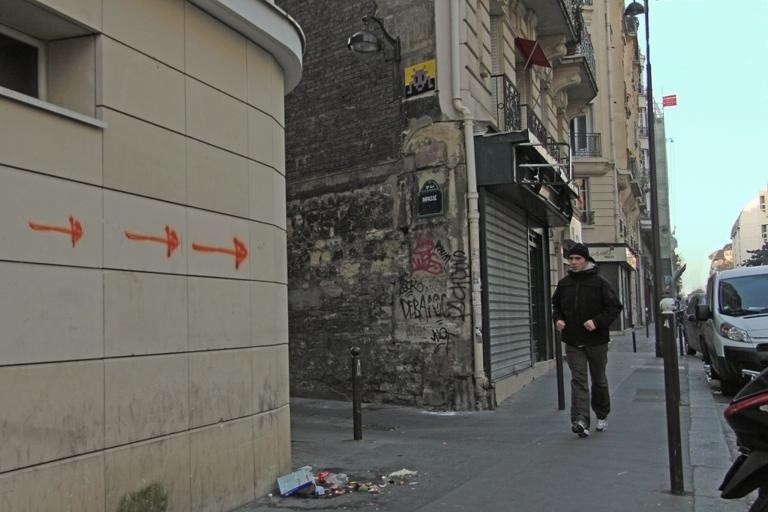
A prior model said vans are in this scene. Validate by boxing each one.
[680,264,768,394]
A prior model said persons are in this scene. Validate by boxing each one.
[550,242,624,438]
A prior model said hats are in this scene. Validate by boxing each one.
[568,243,589,260]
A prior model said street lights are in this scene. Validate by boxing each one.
[623,0,662,355]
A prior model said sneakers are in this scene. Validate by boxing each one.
[596,415,608,432]
[571,420,590,438]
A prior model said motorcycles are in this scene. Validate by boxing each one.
[716,368,768,511]
